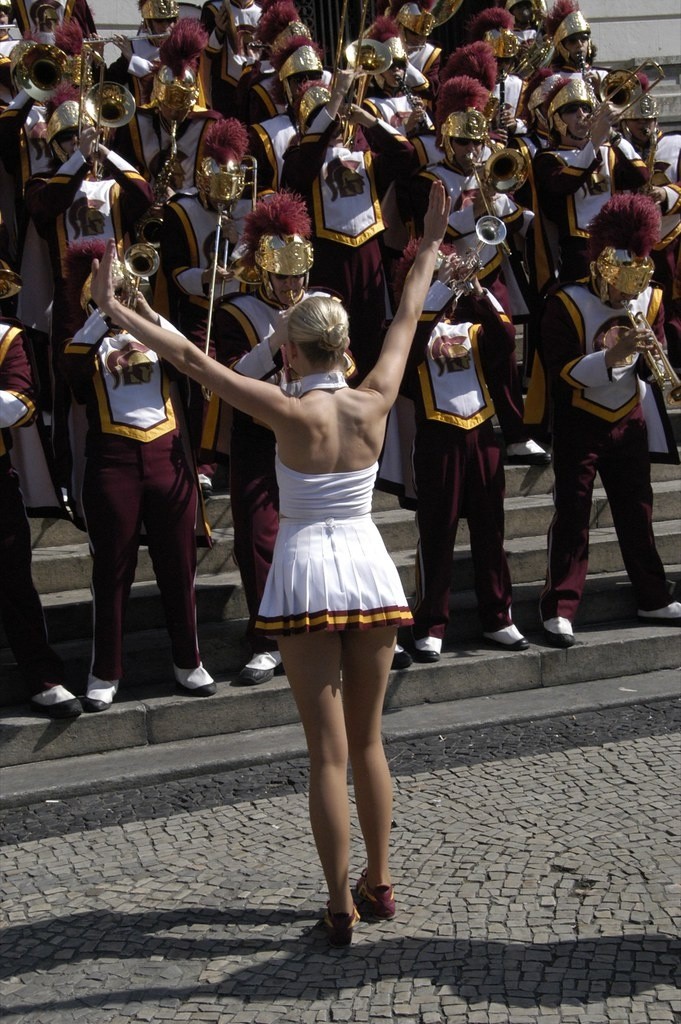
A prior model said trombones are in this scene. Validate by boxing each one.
[201,154,258,403]
[469,136,531,255]
[77,43,137,179]
[328,0,394,152]
[579,57,665,146]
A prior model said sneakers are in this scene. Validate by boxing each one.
[356,868,396,919]
[323,899,361,947]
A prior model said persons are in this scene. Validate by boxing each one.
[86,178,453,949]
[0,0,681,722]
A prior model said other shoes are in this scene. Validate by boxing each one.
[391,644,412,669]
[237,651,282,684]
[415,636,442,661]
[199,473,213,498]
[86,673,119,710]
[542,617,575,647]
[483,624,530,650]
[507,439,552,466]
[31,685,83,719]
[637,601,681,627]
[173,662,217,697]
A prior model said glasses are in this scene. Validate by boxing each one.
[387,61,405,69]
[290,72,322,82]
[274,273,306,280]
[57,130,82,143]
[559,103,592,114]
[566,33,590,43]
[151,17,177,23]
[452,137,481,146]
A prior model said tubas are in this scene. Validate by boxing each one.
[16,43,73,103]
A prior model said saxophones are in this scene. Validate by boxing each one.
[637,127,659,197]
[137,119,181,248]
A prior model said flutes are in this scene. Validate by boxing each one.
[395,76,429,131]
[497,69,507,149]
[82,33,172,44]
[0,24,19,27]
[579,55,596,113]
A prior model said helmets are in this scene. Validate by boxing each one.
[0,0,660,314]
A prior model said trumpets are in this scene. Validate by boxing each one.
[438,215,508,308]
[285,290,296,311]
[110,243,160,333]
[620,299,681,406]
